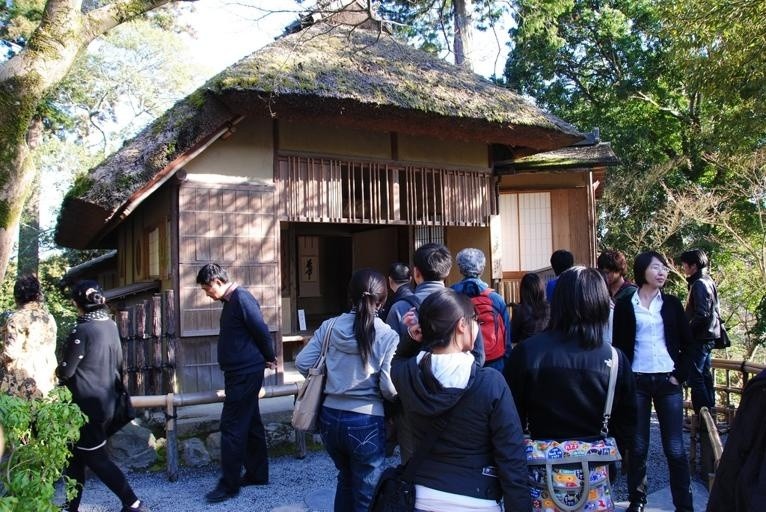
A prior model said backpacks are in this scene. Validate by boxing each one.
[464,282,506,363]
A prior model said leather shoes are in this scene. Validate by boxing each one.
[205,472,267,501]
[625,501,646,512]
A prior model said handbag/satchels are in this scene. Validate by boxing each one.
[714,319,732,349]
[108,375,135,437]
[523,437,623,512]
[290,365,325,432]
[367,467,416,512]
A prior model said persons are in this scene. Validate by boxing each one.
[294,267,401,512]
[390,287,534,510]
[682,249,723,412]
[54,279,145,510]
[501,264,637,510]
[388,245,512,468]
[707,366,766,511]
[511,273,550,345]
[614,250,703,511]
[597,251,637,473]
[196,262,278,503]
[0,277,58,406]
[546,250,575,303]
